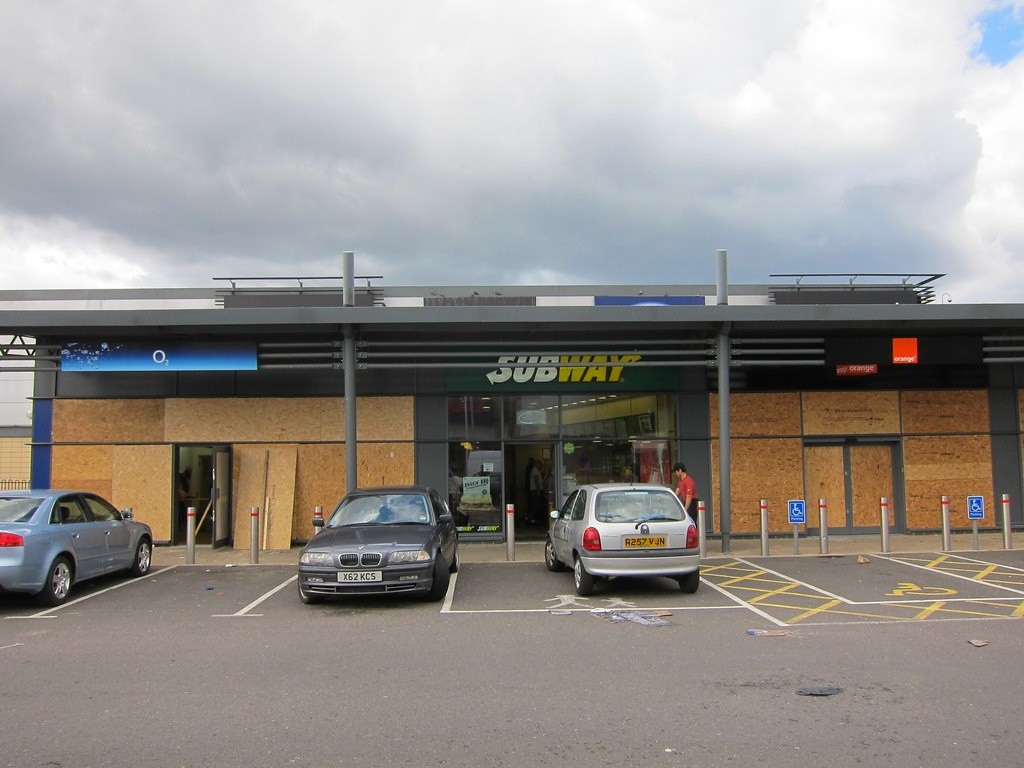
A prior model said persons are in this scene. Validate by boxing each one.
[672,462,698,528]
[523,457,543,526]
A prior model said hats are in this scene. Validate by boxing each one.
[671,463,685,473]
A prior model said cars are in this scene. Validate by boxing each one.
[449,455,501,504]
[562,470,631,512]
[297,486,459,603]
[545,482,701,594]
[0,491,155,607]
